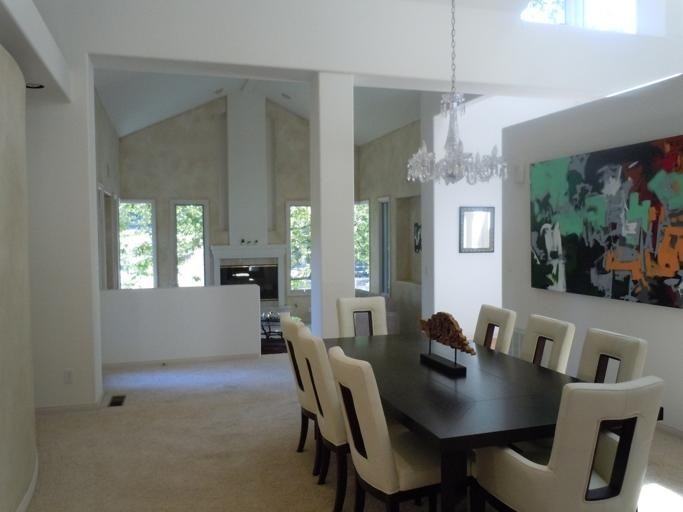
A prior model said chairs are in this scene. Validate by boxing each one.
[518,313,576,374]
[280,316,312,452]
[327,346,444,511]
[575,327,650,383]
[473,303,517,354]
[337,296,389,338]
[473,375,665,512]
[296,326,351,486]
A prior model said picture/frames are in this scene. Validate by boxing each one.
[459,206,494,253]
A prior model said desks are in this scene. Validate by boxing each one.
[320,335,663,510]
[261,305,292,344]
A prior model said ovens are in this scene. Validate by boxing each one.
[220,257,278,300]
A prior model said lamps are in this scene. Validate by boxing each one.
[405,1,527,184]
